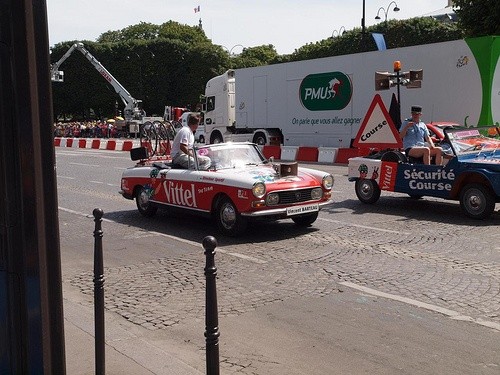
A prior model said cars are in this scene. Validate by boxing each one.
[426,122,500,159]
[118,141,335,235]
[347,124,500,220]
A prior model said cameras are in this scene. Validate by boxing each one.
[408,118,414,122]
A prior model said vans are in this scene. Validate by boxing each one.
[170,112,205,143]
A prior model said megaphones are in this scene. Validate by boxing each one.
[273,162,300,178]
[375,69,423,91]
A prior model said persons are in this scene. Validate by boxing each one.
[170,114,212,170]
[398,105,444,166]
[52,120,119,138]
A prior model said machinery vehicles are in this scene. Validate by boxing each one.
[50,41,190,137]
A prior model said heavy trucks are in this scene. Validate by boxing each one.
[201,36,500,144]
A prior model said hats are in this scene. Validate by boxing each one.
[411,107,421,112]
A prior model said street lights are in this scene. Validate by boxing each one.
[214,44,246,69]
[374,1,400,49]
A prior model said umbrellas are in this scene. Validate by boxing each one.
[116,116,124,121]
[107,119,115,123]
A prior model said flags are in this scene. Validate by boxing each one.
[372,32,387,51]
